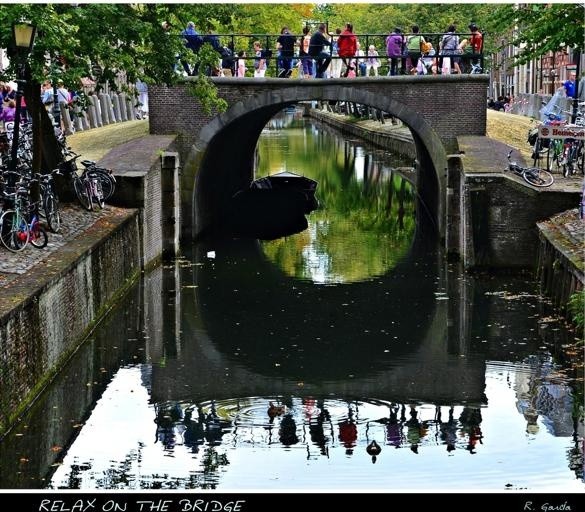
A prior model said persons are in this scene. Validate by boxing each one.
[486,92,511,112]
[0,78,104,132]
[136,79,149,121]
[577,72,585,103]
[563,71,576,123]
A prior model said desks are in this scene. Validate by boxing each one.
[5,21,38,208]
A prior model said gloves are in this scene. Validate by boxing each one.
[232,171,318,213]
